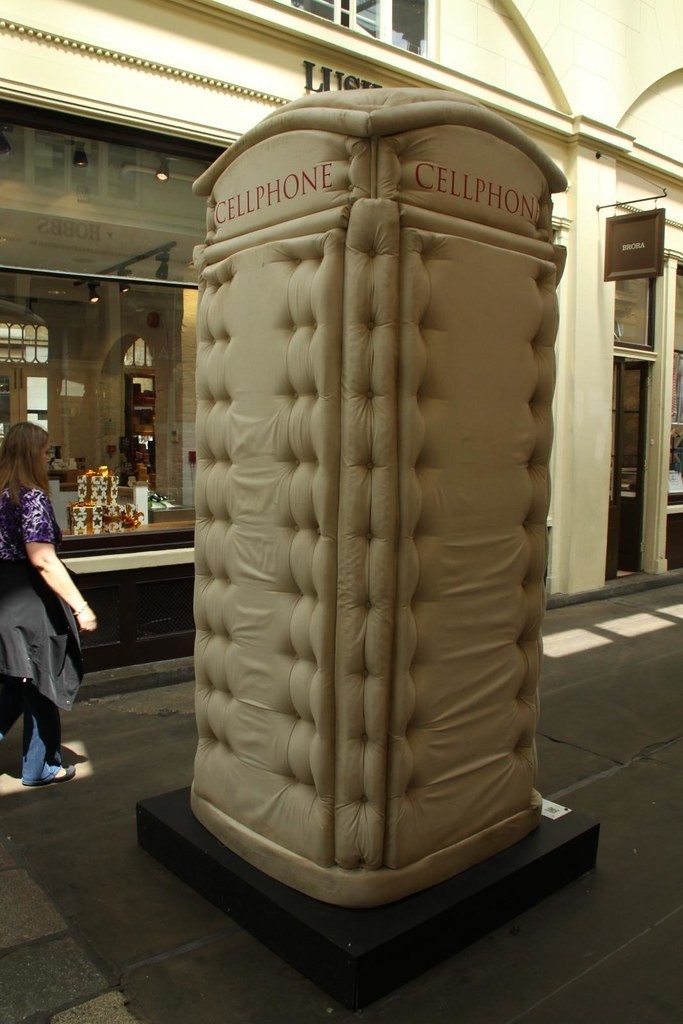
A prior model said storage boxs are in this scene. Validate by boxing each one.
[66,505,103,534]
[77,474,119,506]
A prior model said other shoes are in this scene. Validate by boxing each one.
[22,765,76,785]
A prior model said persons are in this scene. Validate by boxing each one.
[0,422,96,786]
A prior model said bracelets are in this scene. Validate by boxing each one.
[74,601,89,616]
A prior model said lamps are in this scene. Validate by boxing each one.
[69,140,88,166]
[155,253,169,278]
[156,156,170,181]
[117,269,132,296]
[88,282,100,302]
[0,123,12,156]
